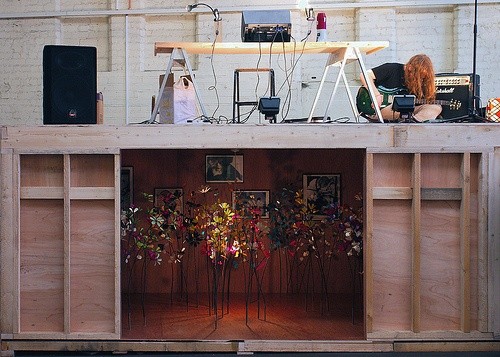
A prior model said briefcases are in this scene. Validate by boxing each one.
[160,74,196,123]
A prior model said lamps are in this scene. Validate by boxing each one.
[391,94,415,123]
[258,96,281,124]
[187,3,221,22]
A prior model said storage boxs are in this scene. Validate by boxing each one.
[161,73,197,123]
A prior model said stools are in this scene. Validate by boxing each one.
[233,67,274,121]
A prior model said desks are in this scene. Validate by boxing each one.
[149,41,389,123]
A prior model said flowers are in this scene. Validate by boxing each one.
[118,186,363,331]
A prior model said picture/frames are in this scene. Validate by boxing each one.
[301,173,342,221]
[204,154,245,183]
[121,166,134,229]
[154,186,185,228]
[230,189,271,219]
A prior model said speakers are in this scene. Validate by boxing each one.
[241,11,291,42]
[42,45,97,123]
[421,72,480,122]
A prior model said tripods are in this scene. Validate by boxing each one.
[424,0,496,123]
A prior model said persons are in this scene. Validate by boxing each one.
[356,54,443,124]
